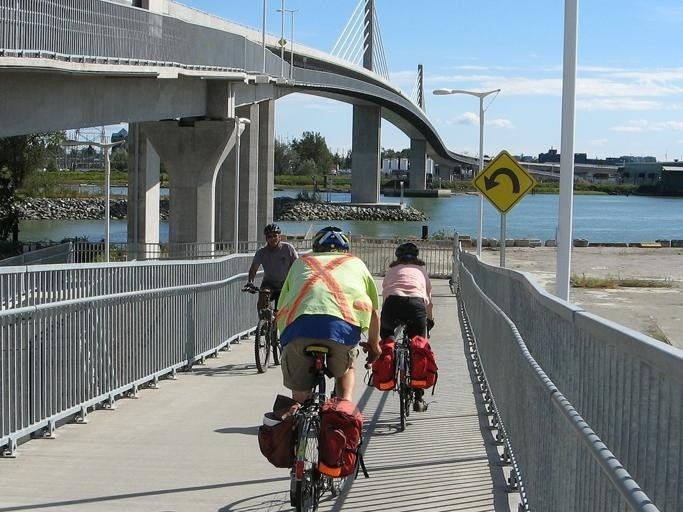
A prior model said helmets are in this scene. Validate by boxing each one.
[263,223,281,234]
[395,243,419,254]
[312,225,350,251]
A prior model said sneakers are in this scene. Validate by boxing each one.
[412,398,429,411]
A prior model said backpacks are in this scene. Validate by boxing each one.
[317,394,365,479]
[407,335,438,390]
[371,338,397,391]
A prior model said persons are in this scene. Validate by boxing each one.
[243,224,299,316]
[257,226,381,478]
[379,241,434,412]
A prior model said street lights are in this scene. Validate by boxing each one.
[276,8,296,80]
[433,88,501,259]
[234,116,251,254]
[62,140,125,262]
